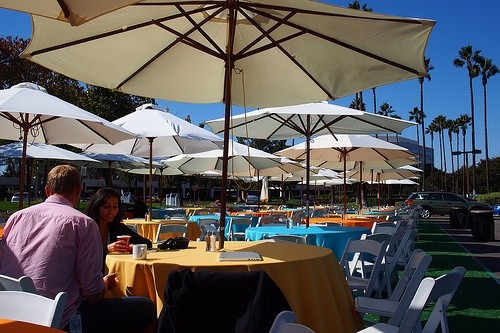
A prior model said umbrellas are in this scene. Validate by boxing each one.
[0,83,424,219]
[0,0,144,27]
[19,0,437,250]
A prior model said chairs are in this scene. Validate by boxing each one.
[0,203,467,333]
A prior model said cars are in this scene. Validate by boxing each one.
[405,191,477,219]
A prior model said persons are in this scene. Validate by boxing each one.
[123,192,148,218]
[0,164,157,333]
[80,187,152,278]
[186,192,191,200]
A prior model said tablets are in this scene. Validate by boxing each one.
[218,252,262,261]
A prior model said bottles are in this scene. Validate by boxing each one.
[145,213,150,222]
[287,218,293,228]
[205,234,216,252]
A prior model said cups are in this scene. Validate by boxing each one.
[116,235,131,252]
[133,244,147,260]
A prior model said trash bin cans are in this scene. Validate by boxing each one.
[451,206,466,228]
[471,210,495,241]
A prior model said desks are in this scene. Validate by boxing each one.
[304,217,381,227]
[246,227,374,276]
[104,241,364,333]
[187,215,250,240]
[121,218,202,242]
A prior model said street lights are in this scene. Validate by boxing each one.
[451,150,482,193]
